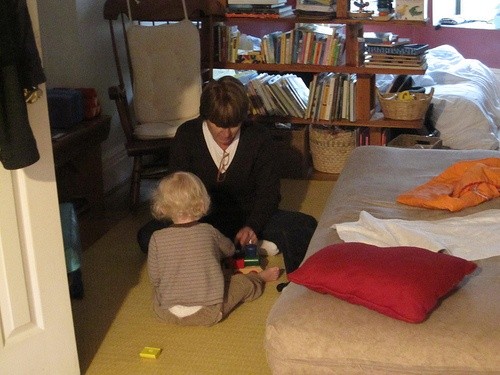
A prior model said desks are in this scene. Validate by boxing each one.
[50,115,127,246]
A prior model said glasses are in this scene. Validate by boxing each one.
[217,150,229,182]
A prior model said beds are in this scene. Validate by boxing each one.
[266,145,500,375]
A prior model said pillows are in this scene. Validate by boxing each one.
[288,243,477,323]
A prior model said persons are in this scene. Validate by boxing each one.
[147,172,280,328]
[136,74,318,292]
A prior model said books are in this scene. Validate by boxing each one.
[212,69,358,123]
[213,22,347,66]
[225,0,294,18]
[293,0,336,19]
[356,31,429,71]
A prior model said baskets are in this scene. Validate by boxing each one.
[376,86,434,120]
[309,127,359,173]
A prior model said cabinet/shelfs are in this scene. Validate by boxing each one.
[103,0,439,180]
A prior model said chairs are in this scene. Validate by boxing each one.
[108,21,218,207]
